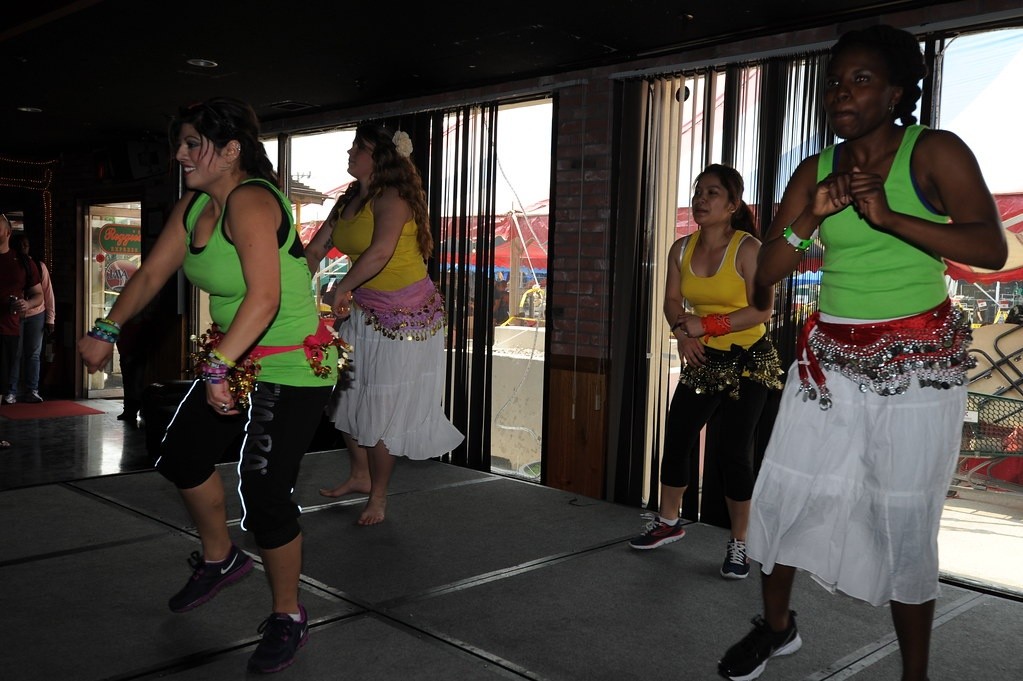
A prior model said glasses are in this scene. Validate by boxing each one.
[0,208,9,223]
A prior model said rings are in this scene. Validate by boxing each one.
[684,332,687,335]
[221,404,227,412]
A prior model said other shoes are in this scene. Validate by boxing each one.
[117,409,137,420]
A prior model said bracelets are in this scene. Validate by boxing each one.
[670,323,682,332]
[196,348,237,385]
[87,317,122,344]
[783,218,814,253]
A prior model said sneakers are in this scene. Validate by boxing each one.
[626,513,685,550]
[717,610,802,681]
[247,603,311,674]
[4,394,17,403]
[167,544,253,613]
[720,538,751,580]
[25,390,43,403]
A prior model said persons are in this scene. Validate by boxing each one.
[319,276,352,318]
[717,25,1009,681]
[305,123,466,526]
[117,290,167,422]
[441,269,546,332]
[0,216,55,403]
[76,98,354,672]
[629,164,784,579]
